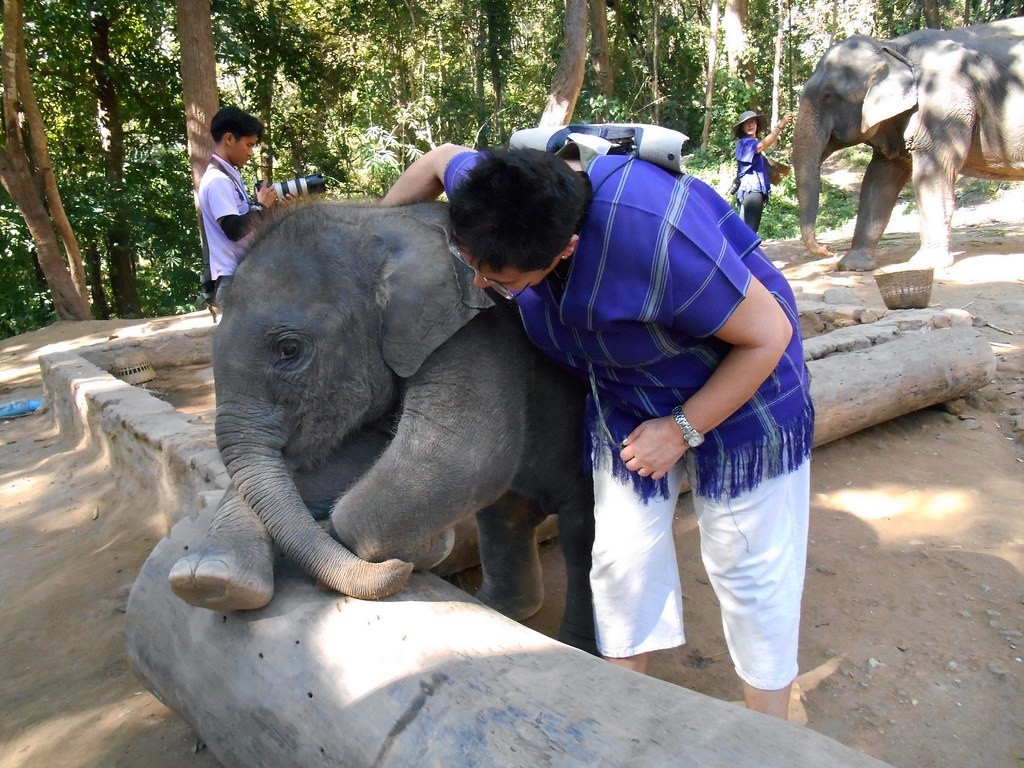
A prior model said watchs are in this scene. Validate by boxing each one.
[672,404,706,449]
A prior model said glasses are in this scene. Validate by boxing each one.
[448,234,571,302]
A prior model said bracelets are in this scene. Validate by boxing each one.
[776,126,783,132]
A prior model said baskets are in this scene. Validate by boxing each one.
[874,267,934,310]
[766,156,791,186]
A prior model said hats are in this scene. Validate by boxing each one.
[731,110,767,137]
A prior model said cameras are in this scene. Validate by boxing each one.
[255,173,326,200]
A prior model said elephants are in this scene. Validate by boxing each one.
[167,194,601,658]
[793,17,1024,271]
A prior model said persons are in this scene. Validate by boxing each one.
[198,105,301,312]
[733,112,792,234]
[378,143,817,722]
